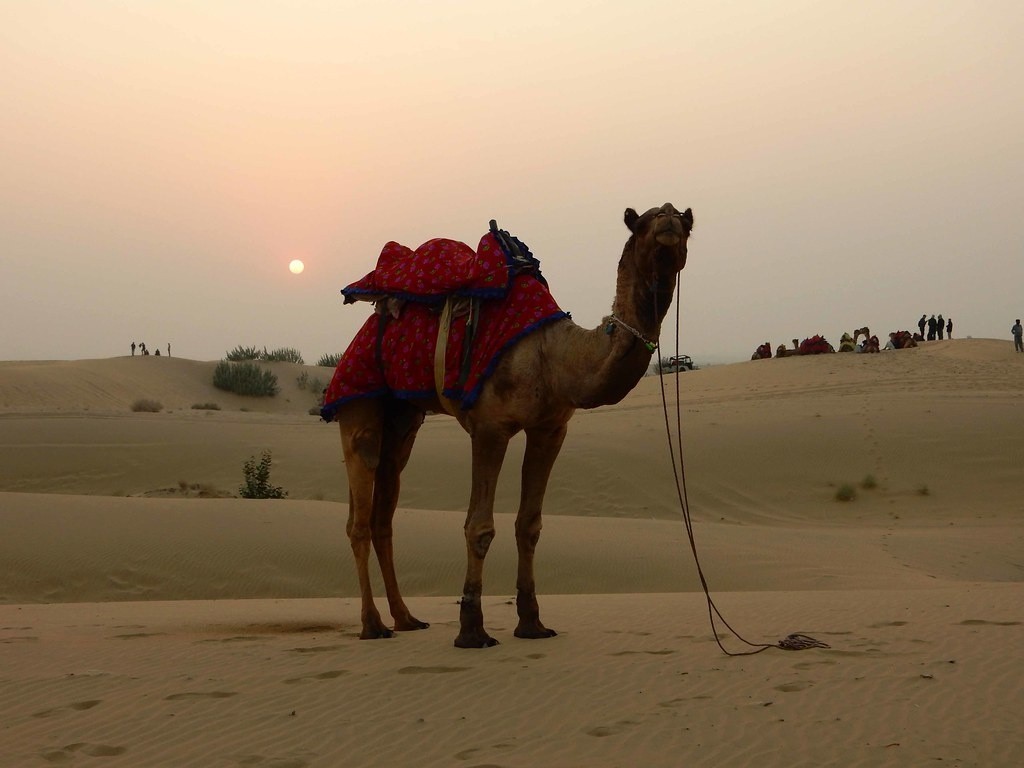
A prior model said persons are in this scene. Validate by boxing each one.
[859,314,953,353]
[1011,319,1024,352]
[131,341,170,357]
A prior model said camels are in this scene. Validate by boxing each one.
[750,326,919,361]
[320,203,693,650]
